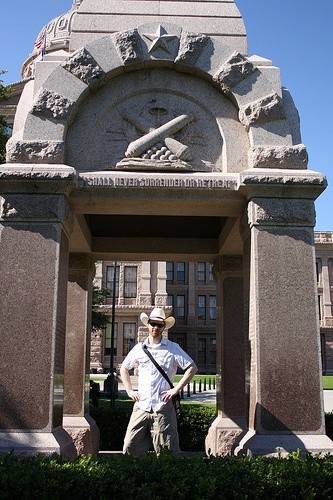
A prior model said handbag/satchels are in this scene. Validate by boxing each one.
[172,392,181,420]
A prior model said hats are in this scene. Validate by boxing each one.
[140,307,176,331]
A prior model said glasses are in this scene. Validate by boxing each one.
[148,322,165,328]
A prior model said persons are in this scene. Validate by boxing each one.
[90,379,100,408]
[120,307,199,459]
[104,369,119,408]
